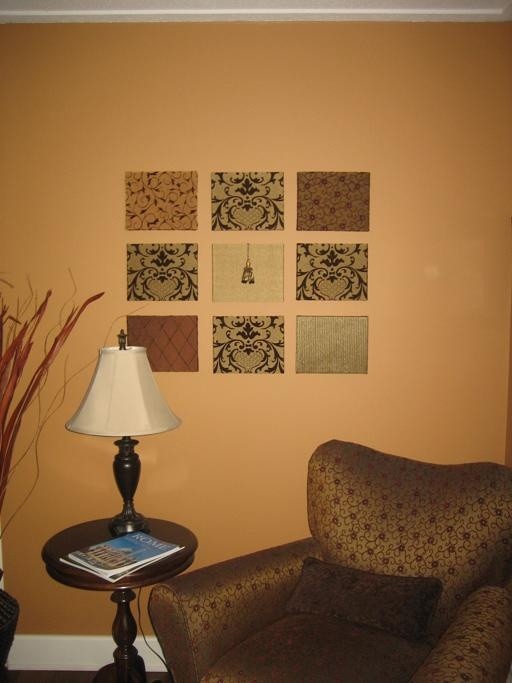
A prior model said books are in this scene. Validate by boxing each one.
[68,530,180,578]
[58,545,189,583]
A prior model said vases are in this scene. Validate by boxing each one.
[0,568,19,683]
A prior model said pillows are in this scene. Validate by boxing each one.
[286,556,444,641]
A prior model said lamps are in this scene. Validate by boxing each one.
[64,329,181,541]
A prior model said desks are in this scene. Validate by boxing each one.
[40,516,198,683]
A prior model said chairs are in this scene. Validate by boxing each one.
[147,440,512,683]
